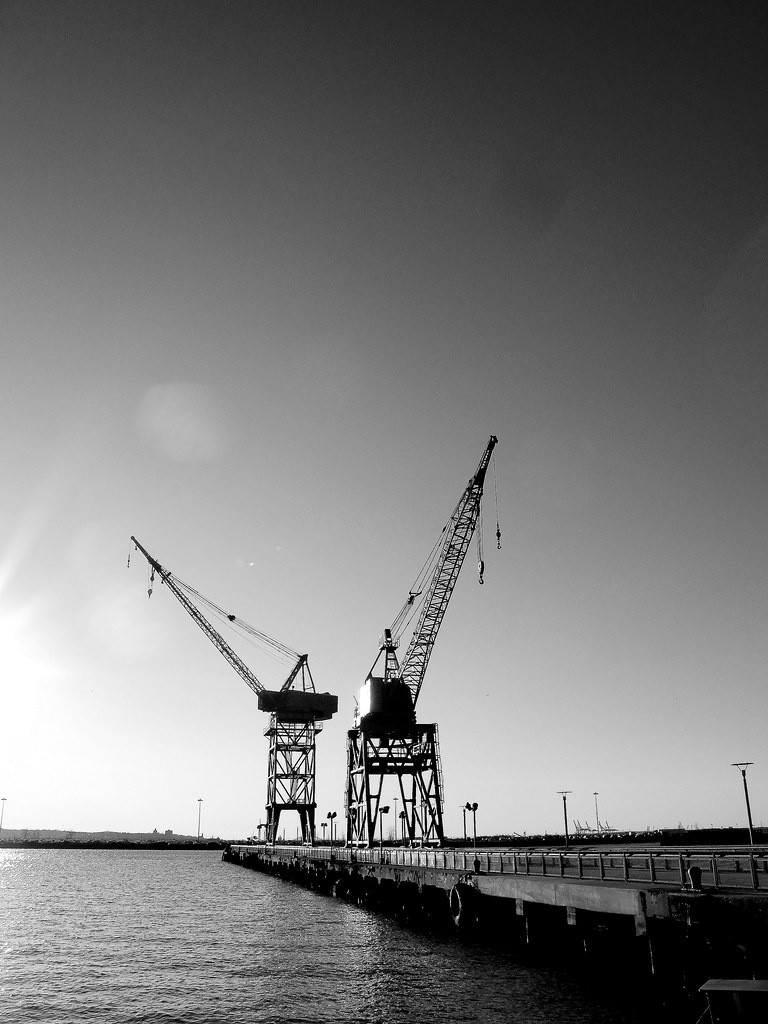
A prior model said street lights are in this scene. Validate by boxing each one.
[399,811,404,846]
[393,797,399,839]
[429,809,436,838]
[379,805,390,863]
[593,792,600,833]
[321,823,327,840]
[465,802,478,847]
[558,792,571,851]
[197,799,203,836]
[732,762,754,845]
[327,812,336,846]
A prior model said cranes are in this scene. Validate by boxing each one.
[342,436,501,848]
[573,820,619,833]
[126,536,334,846]
